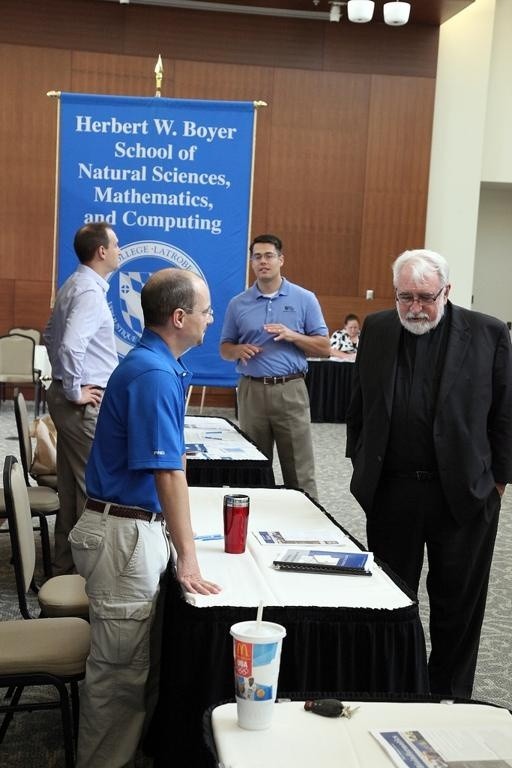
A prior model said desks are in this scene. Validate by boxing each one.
[199,694,512,768]
[32,345,55,415]
[140,484,430,768]
[184,414,276,486]
[305,355,357,424]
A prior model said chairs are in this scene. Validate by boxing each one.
[1,334,43,422]
[3,327,43,402]
[2,455,92,624]
[14,391,59,493]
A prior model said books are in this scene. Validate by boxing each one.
[185,443,208,453]
[271,548,374,576]
[204,431,222,440]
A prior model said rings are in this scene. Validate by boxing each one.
[278,329,280,332]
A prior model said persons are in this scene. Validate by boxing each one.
[219,234,331,504]
[43,221,123,575]
[65,267,224,768]
[344,248,512,700]
[330,314,362,361]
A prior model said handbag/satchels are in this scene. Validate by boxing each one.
[30,415,57,476]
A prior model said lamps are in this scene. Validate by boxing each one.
[346,0,375,24]
[329,1,346,22]
[382,0,412,26]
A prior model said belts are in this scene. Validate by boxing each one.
[85,500,162,521]
[250,375,302,385]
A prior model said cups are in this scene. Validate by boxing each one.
[228,620,287,730]
[366,290,374,300]
[222,494,251,555]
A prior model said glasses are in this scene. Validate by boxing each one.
[394,288,442,305]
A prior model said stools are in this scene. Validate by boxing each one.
[1,486,63,579]
[1,616,95,768]
